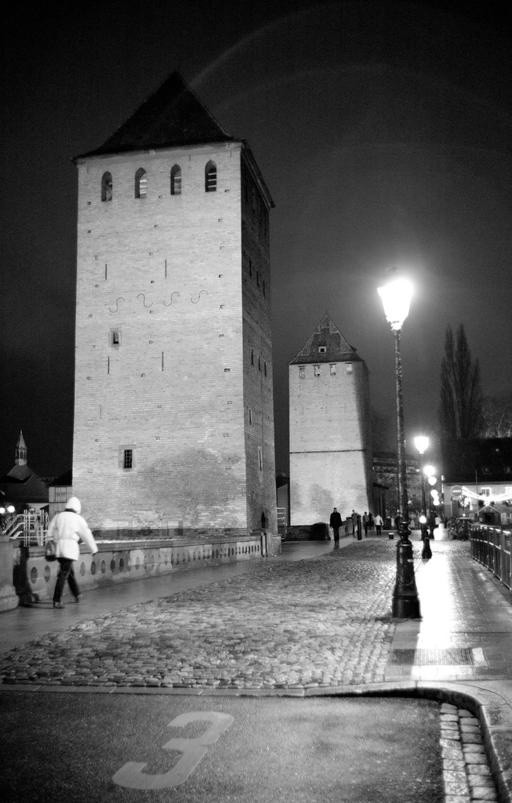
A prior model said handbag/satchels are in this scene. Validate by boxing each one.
[45,539,57,562]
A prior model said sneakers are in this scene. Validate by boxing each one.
[76,593,84,604]
[52,601,66,609]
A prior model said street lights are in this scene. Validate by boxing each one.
[375,275,427,619]
[413,430,443,560]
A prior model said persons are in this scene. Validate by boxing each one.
[330,507,342,544]
[45,496,100,608]
[362,508,450,535]
[351,509,360,536]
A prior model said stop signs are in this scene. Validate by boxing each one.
[451,485,462,496]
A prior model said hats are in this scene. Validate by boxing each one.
[64,496,82,514]
